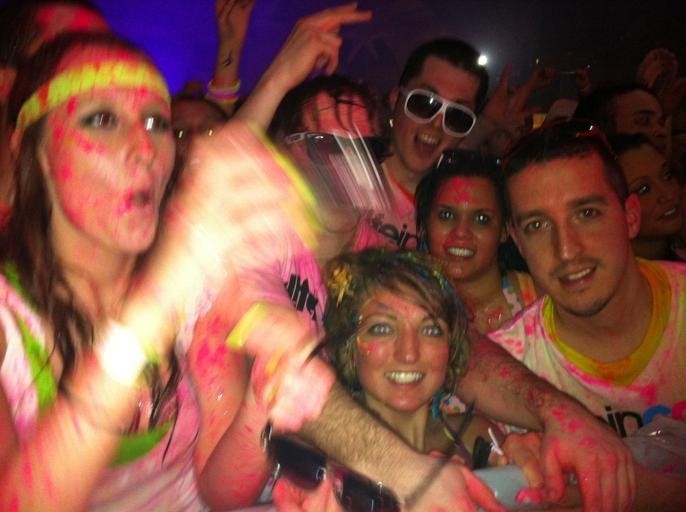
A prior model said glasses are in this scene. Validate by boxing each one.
[281,131,388,165]
[436,148,502,174]
[400,86,477,138]
[259,325,477,512]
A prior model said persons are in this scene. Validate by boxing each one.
[0,0,686,512]
